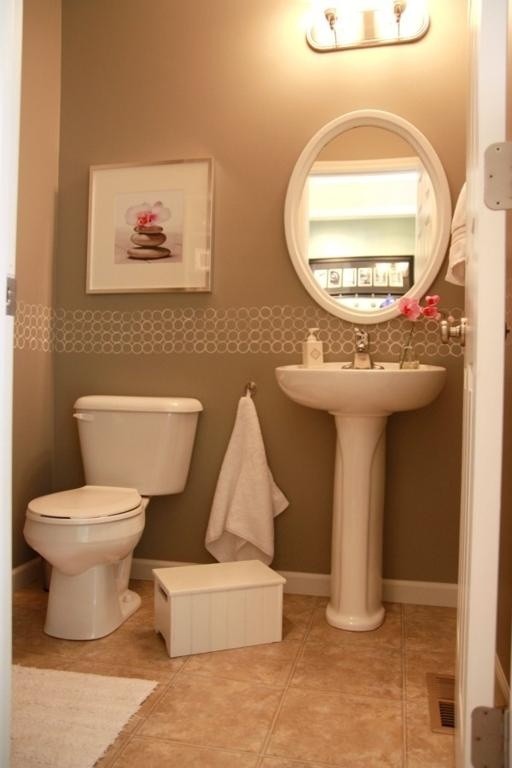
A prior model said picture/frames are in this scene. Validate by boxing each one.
[82,152,217,299]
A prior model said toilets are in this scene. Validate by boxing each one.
[23,393,204,641]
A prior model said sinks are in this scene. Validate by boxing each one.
[275,362,446,415]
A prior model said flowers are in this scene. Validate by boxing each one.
[396,291,442,367]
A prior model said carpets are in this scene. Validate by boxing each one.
[14,658,163,765]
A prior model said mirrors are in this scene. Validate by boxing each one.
[284,107,455,329]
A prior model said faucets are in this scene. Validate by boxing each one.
[352,328,371,369]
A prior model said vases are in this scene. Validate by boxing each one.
[399,342,416,368]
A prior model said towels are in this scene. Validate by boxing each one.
[204,391,293,572]
[447,177,468,289]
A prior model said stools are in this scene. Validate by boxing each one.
[148,559,288,659]
